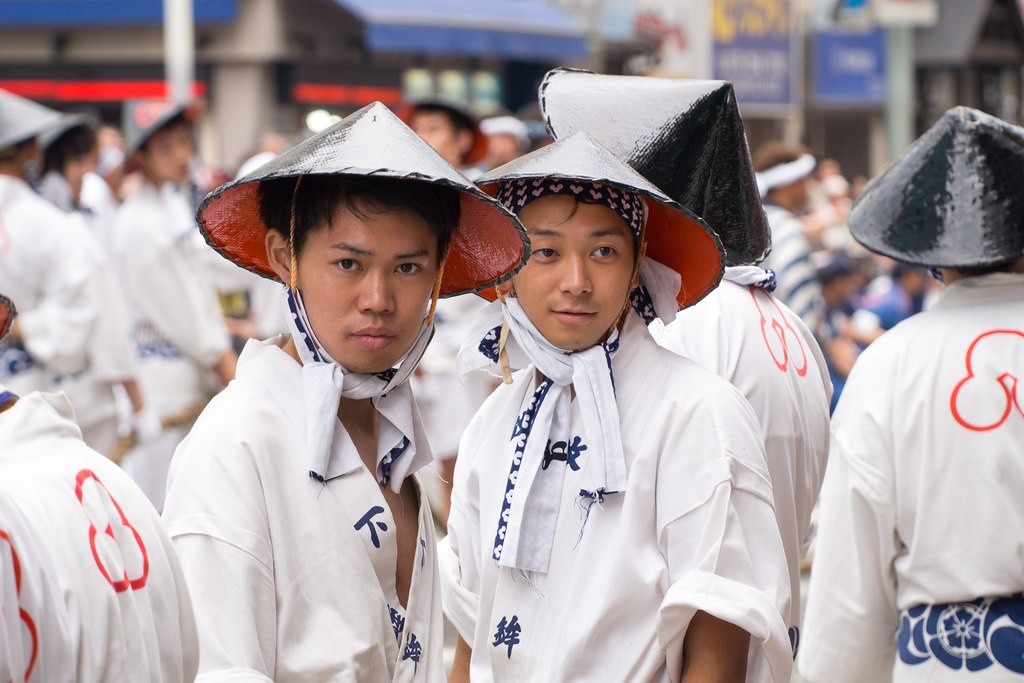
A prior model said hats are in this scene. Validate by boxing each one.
[197,100,531,299]
[0,90,65,151]
[539,65,772,267]
[472,129,727,318]
[847,105,1023,266]
[39,104,100,152]
[121,98,190,165]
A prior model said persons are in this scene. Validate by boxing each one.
[1,387,200,683]
[746,135,951,425]
[157,100,460,683]
[436,127,792,683]
[793,106,1024,682]
[0,81,293,519]
[539,66,828,674]
[398,100,557,536]
